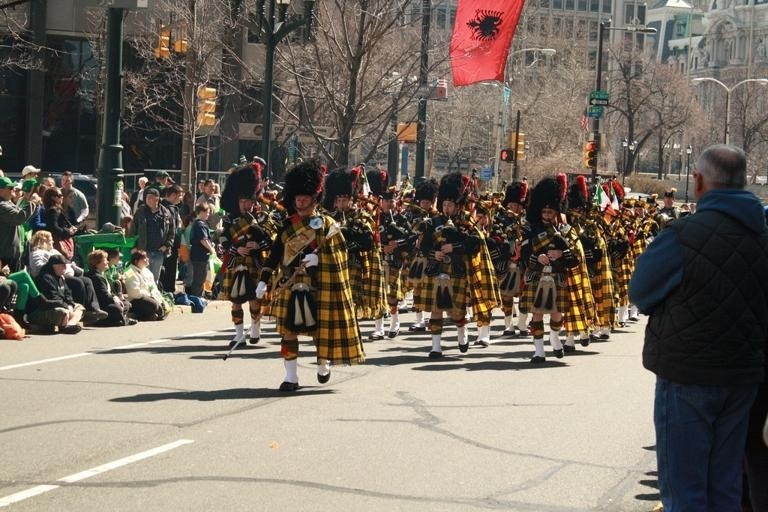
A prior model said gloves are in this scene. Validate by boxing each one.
[302,254,319,267]
[256,281,267,298]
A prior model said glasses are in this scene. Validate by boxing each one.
[56,194,64,198]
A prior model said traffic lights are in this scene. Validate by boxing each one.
[191,82,217,129]
[175,40,189,54]
[153,22,170,60]
[511,133,526,162]
[500,150,514,162]
[583,141,595,170]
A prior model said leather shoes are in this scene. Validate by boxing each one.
[64,325,81,334]
[530,315,640,363]
[409,326,425,331]
[249,337,259,344]
[479,340,489,346]
[388,329,399,338]
[520,330,529,336]
[369,333,384,339]
[228,340,246,347]
[127,318,138,325]
[503,331,515,335]
[317,369,330,383]
[279,381,298,392]
[459,342,469,352]
[429,351,442,358]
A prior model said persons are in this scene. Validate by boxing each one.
[628,142,767,512]
[0,153,698,391]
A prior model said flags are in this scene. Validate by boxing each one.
[449,1,525,88]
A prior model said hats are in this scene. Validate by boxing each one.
[221,166,413,213]
[0,177,18,188]
[646,196,657,204]
[633,200,645,207]
[664,189,676,197]
[155,171,169,176]
[22,179,39,192]
[48,255,68,264]
[569,175,587,208]
[22,164,40,177]
[417,173,566,213]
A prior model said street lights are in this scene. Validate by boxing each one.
[628,141,634,174]
[621,136,628,186]
[689,76,768,149]
[229,0,315,183]
[583,18,657,183]
[683,145,694,203]
[378,70,417,188]
[493,48,556,191]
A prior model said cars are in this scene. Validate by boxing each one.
[44,169,96,212]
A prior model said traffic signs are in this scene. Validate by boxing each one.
[589,98,608,107]
[586,112,604,118]
[589,90,609,99]
[586,106,605,113]
